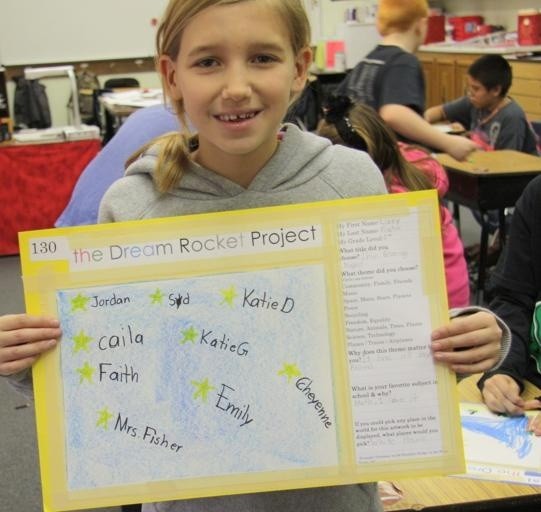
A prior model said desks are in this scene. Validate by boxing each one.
[377,364,541,512]
[429,148,541,293]
[97,86,174,138]
[1,137,102,255]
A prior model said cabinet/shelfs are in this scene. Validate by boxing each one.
[505,61,541,127]
[415,32,484,129]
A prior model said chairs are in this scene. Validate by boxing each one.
[105,78,140,141]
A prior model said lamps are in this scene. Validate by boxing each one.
[23,61,102,142]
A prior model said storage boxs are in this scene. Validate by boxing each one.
[518,9,541,45]
[424,13,446,45]
[449,15,483,40]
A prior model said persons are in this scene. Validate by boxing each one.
[346,1,541,287]
[52,102,196,231]
[0,0,511,511]
[477,174,541,433]
[317,94,471,308]
